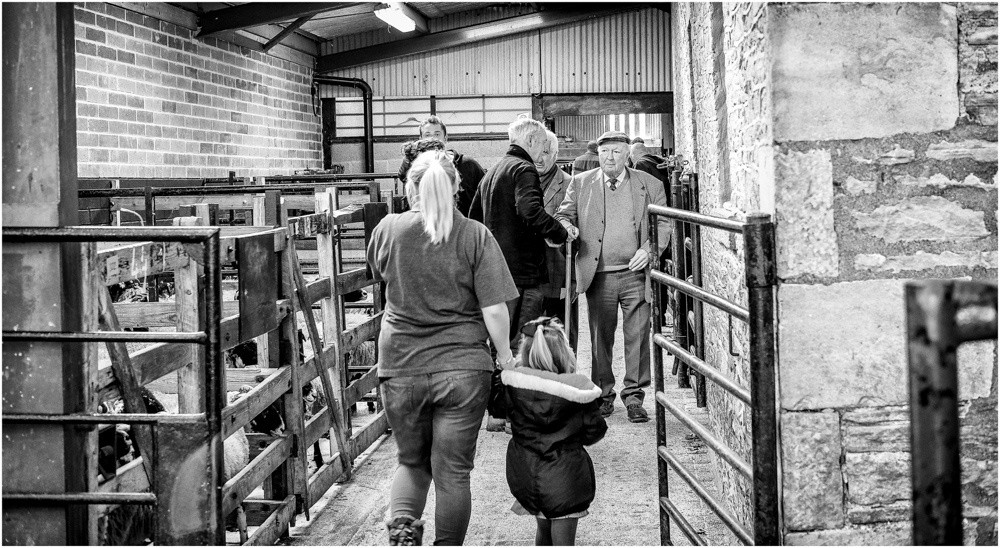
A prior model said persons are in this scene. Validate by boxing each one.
[399,118,671,423]
[487,314,608,547]
[366,151,520,546]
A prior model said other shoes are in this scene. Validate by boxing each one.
[386,516,425,546]
[487,416,512,434]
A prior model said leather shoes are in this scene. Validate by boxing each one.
[600,402,614,418]
[627,404,648,421]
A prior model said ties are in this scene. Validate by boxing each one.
[609,179,617,190]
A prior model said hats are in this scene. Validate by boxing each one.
[596,131,631,146]
[633,137,644,144]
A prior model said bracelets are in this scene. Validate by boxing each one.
[496,349,513,365]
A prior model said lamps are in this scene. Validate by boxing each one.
[372,2,417,34]
